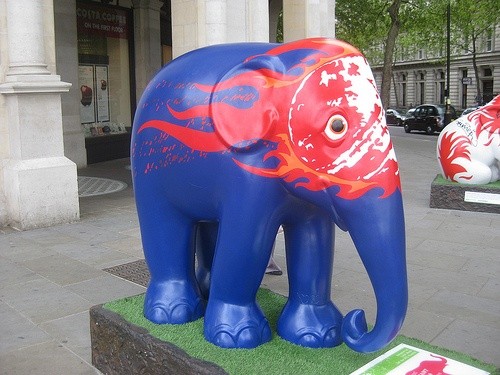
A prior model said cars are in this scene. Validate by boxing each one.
[404,103,457,135]
[386,108,406,126]
[462,106,481,116]
[408,109,418,115]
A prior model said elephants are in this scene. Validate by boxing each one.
[130,37,409,354]
